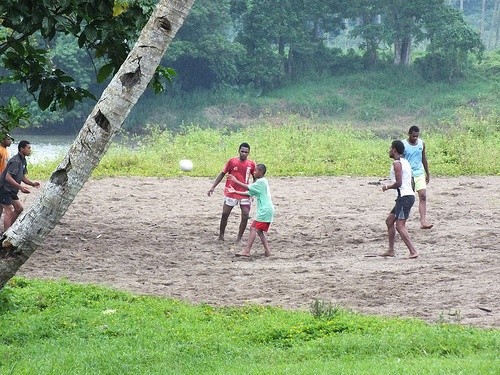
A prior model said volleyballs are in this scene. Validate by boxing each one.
[180,160,193,171]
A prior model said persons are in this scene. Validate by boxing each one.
[0,140,40,232]
[400,125,434,230]
[378,140,418,259]
[224,163,274,256]
[0,134,15,219]
[207,142,258,244]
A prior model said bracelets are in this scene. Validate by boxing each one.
[385,185,388,190]
